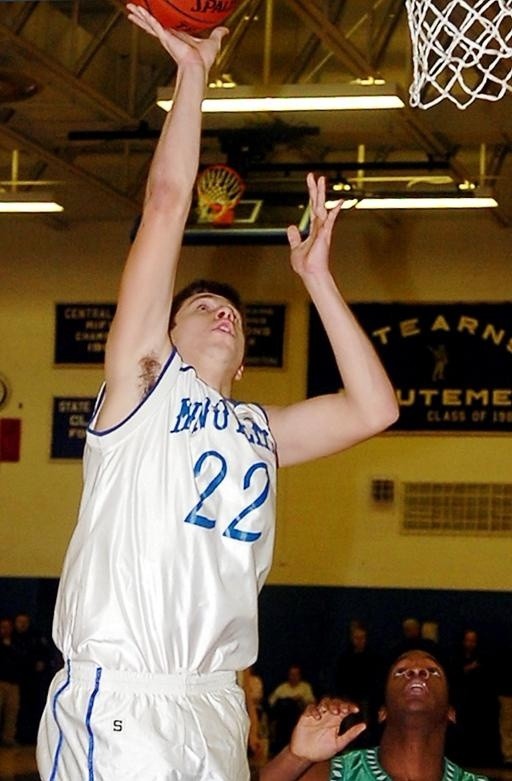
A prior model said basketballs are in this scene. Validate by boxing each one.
[127,2,239,35]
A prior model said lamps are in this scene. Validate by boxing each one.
[154,0,409,116]
[323,140,501,212]
[0,151,69,215]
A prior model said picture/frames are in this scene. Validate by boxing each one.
[49,297,117,372]
[46,392,97,462]
[305,296,511,439]
[238,301,293,371]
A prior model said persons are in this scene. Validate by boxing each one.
[2,603,512,781]
[34,2,400,781]
